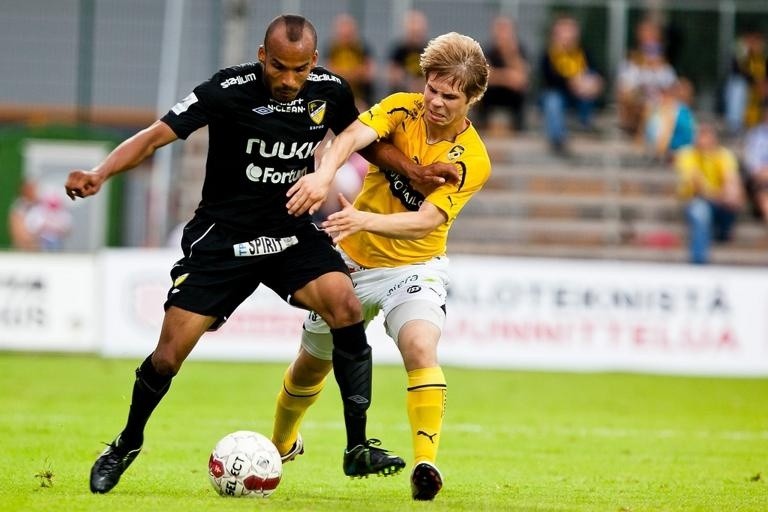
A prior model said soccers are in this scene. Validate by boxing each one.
[210,431,281,497]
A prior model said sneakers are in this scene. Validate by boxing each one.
[281,430,305,465]
[409,460,444,502]
[342,438,407,481]
[89,430,145,495]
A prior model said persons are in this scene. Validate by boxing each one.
[268,31,492,499]
[65,16,463,492]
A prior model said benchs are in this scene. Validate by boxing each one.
[445,95,767,264]
